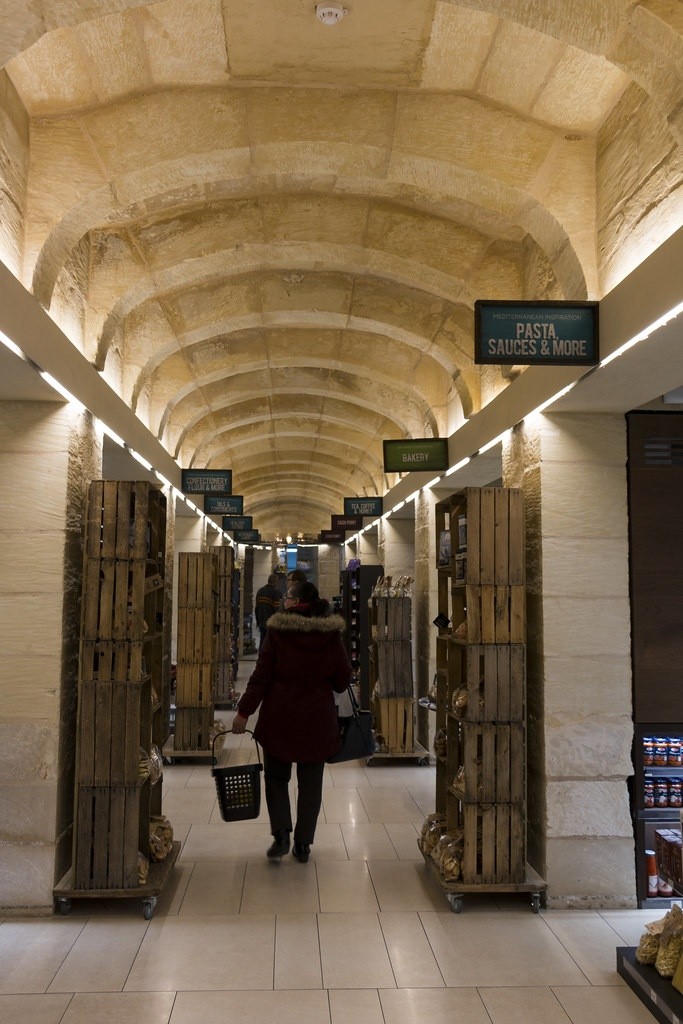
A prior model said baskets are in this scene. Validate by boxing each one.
[210,763,263,822]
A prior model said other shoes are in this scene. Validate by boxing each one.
[292,842,312,863]
[267,835,290,858]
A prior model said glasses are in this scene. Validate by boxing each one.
[282,595,297,601]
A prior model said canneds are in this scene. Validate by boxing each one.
[642,737,683,810]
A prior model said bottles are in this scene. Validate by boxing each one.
[645,850,658,897]
[658,878,674,897]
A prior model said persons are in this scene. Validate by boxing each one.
[230,581,349,863]
[254,573,281,656]
[277,570,305,616]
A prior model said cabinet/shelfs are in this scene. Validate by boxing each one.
[417,485,549,913]
[158,551,227,764]
[348,565,385,724]
[207,545,241,708]
[615,721,682,1023]
[331,564,358,683]
[52,478,183,920]
[365,596,429,768]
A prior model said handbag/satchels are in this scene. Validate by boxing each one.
[325,684,376,764]
